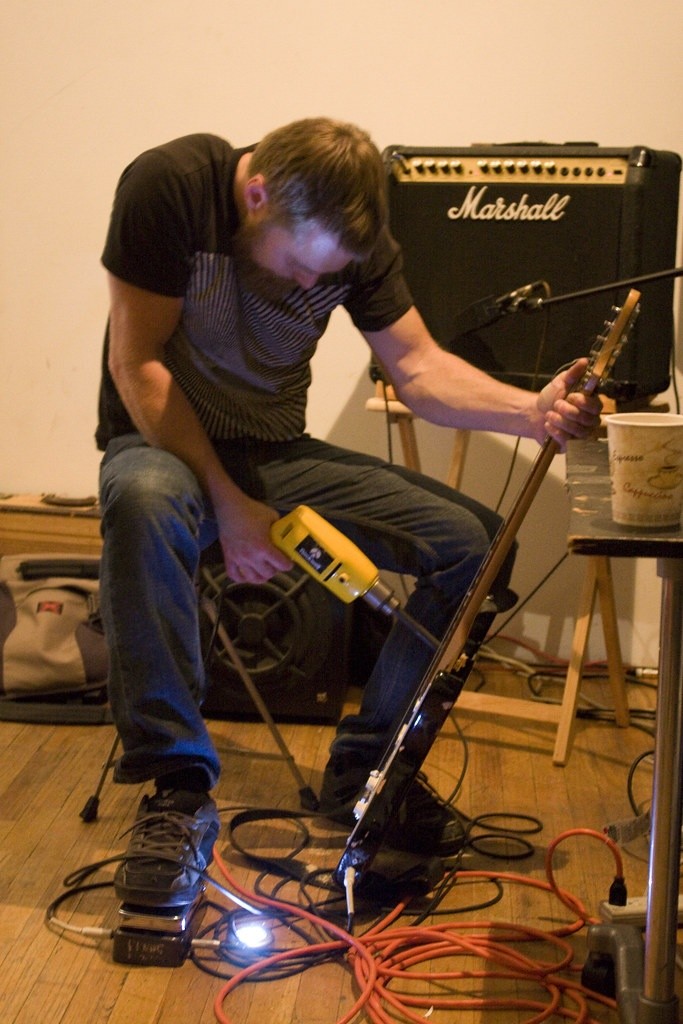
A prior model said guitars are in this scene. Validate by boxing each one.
[330,286,644,894]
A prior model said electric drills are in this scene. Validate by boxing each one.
[271,504,441,651]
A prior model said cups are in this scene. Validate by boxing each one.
[605,413,683,529]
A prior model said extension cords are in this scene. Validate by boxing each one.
[598,896,683,927]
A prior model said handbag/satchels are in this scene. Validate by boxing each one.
[0,551,114,725]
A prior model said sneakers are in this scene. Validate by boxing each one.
[114,793,220,907]
[318,756,466,856]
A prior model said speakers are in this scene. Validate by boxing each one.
[369,144,683,397]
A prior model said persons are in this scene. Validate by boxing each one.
[95,117,605,910]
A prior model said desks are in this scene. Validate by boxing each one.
[363,397,629,766]
[562,437,683,1024]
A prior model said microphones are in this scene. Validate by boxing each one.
[494,282,545,315]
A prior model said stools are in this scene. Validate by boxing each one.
[76,544,320,821]
